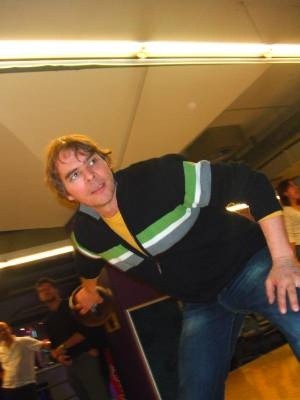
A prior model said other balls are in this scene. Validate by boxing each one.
[69,283,115,327]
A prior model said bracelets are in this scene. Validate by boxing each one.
[56,345,66,351]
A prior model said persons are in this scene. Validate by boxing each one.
[277,179,300,261]
[37,278,112,400]
[0,321,52,400]
[44,132,300,400]
